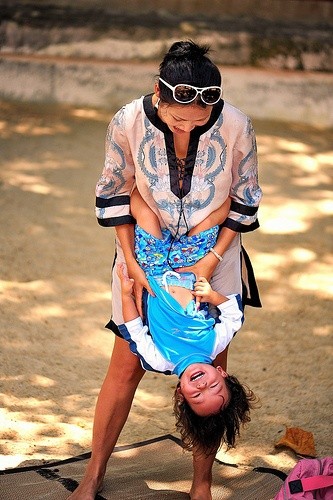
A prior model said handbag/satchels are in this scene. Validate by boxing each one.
[274,457,333,500]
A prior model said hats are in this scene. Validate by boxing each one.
[158,77,223,105]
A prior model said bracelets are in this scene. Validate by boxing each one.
[210,248,223,262]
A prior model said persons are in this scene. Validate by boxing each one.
[66,41,267,500]
[116,188,263,460]
